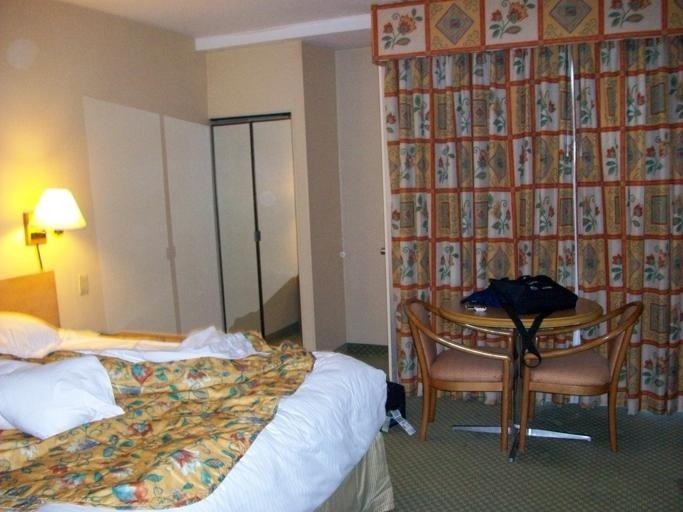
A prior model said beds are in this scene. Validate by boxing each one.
[1,270,408,512]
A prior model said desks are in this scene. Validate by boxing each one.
[435,292,606,466]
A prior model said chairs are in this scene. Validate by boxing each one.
[401,294,521,456]
[516,296,649,454]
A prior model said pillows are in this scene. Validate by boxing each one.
[1,350,130,443]
[2,300,62,364]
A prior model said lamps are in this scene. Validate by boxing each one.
[20,183,93,273]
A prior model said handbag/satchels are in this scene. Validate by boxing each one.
[488,275,577,314]
